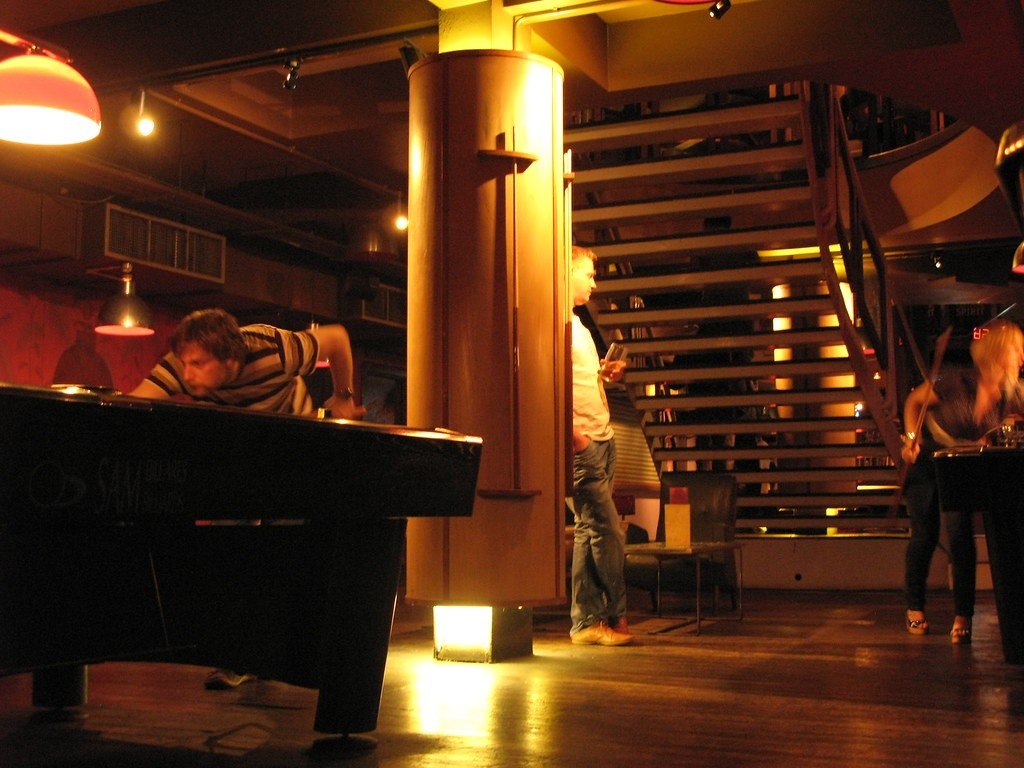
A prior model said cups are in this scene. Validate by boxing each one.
[599,342,628,382]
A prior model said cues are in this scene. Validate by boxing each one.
[504,116,527,490]
[885,326,956,531]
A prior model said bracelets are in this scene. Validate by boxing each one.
[333,388,353,400]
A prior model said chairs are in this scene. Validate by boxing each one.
[625,468,741,614]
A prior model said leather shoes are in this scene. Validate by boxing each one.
[608,616,630,634]
[572,618,635,646]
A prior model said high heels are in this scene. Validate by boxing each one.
[904,607,930,635]
[949,613,973,644]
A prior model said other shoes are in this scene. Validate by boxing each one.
[204,667,259,690]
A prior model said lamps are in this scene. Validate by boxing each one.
[0,21,101,148]
[86,262,156,336]
[707,0,731,20]
[346,219,398,264]
[50,324,115,393]
[281,56,302,90]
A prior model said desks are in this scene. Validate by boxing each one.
[932,442,1023,664]
[626,540,746,635]
[0,384,481,757]
[622,543,744,633]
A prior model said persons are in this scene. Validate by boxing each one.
[568,247,639,646]
[127,308,366,689]
[900,317,1024,666]
[678,217,761,470]
